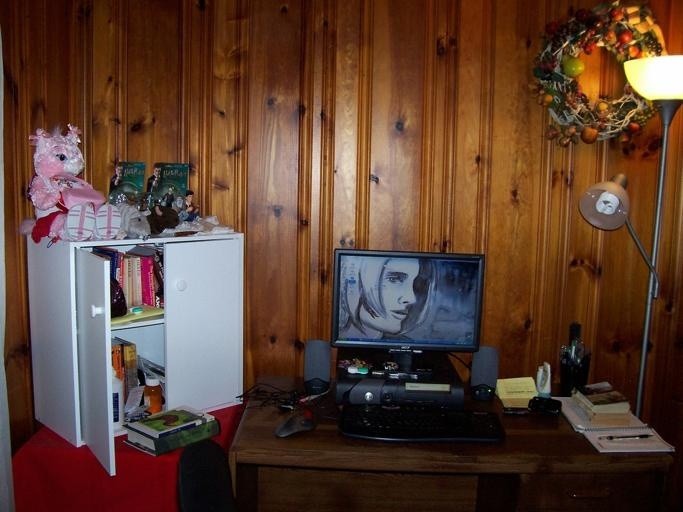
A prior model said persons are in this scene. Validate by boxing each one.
[334,255,450,343]
[178,189,201,224]
[150,198,177,231]
[108,158,146,196]
[145,160,189,195]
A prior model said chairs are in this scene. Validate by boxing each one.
[177,441,234,511]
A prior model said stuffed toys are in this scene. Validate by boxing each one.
[160,186,174,210]
[113,202,151,239]
[27,125,118,241]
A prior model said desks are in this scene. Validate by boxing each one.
[229,385,674,512]
[12,405,245,511]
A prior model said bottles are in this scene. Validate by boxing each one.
[143,375,162,413]
[536,364,552,397]
[558,320,586,396]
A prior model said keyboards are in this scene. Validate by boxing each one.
[337,403,505,443]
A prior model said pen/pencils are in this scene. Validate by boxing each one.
[571,340,576,361]
[597,435,655,440]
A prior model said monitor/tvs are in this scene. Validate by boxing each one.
[330,249,486,376]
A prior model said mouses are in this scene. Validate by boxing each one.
[274,410,314,437]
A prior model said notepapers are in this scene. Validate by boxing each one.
[495,376,539,408]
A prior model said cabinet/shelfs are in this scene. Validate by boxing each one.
[26,225,246,477]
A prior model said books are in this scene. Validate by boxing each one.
[86,245,165,416]
[553,381,678,454]
[496,372,539,409]
[120,419,220,456]
[127,404,214,439]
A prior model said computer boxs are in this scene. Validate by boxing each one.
[335,346,465,408]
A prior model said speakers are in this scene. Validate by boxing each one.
[304,340,331,392]
[472,347,497,399]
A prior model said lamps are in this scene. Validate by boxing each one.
[579,54,683,418]
